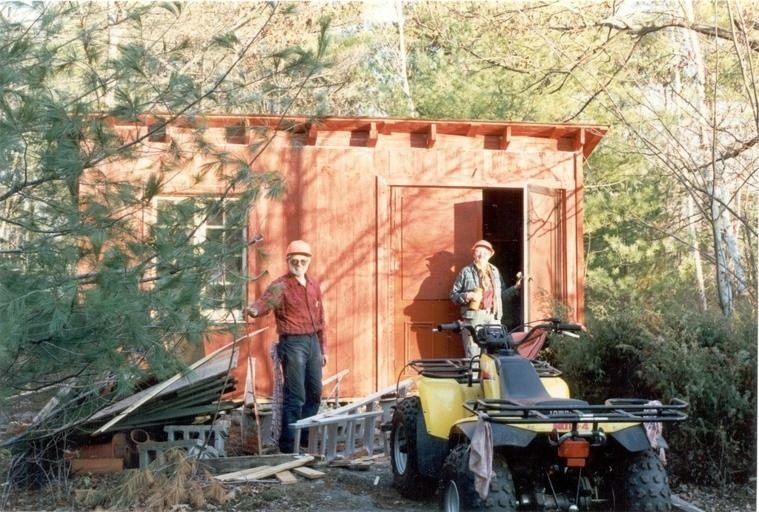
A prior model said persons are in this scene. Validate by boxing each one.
[450,240,523,378]
[240,240,329,453]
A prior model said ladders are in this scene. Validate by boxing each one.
[287,378,415,467]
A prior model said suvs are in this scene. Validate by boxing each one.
[390,318,691,512]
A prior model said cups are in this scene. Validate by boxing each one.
[469,284,483,311]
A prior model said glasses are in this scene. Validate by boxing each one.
[290,259,308,265]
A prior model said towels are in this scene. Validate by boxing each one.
[643,399,670,467]
[265,341,285,449]
[466,411,497,500]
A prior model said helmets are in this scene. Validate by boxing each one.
[470,240,495,255]
[285,240,312,256]
